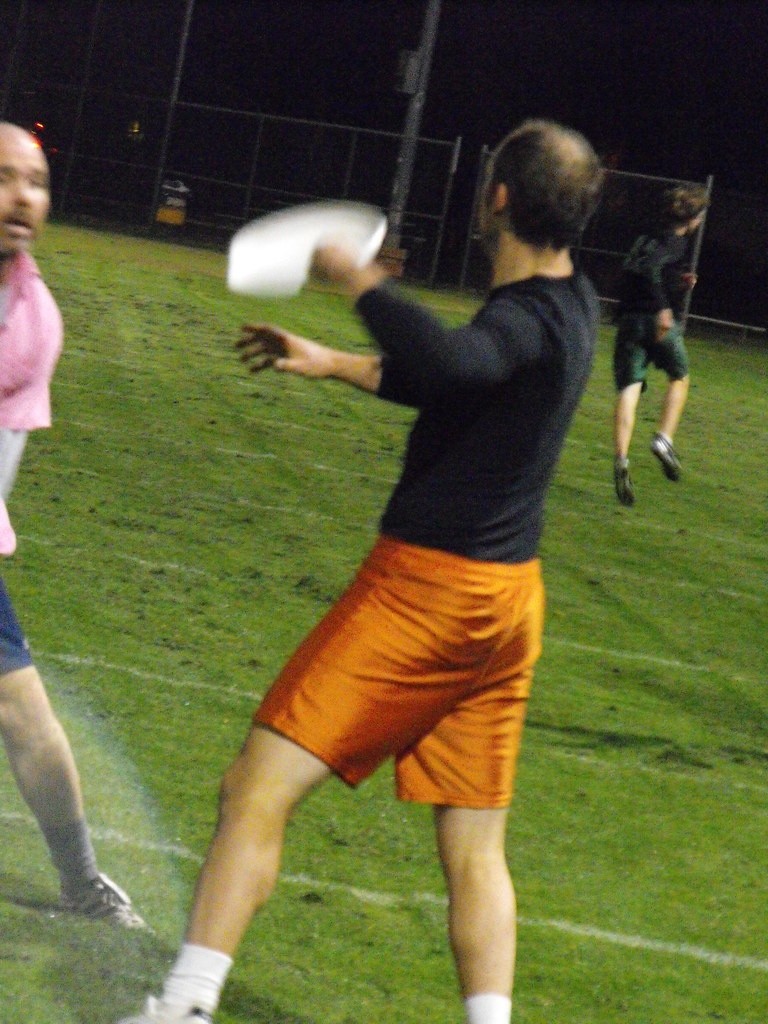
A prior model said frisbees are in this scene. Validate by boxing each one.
[225,199,387,300]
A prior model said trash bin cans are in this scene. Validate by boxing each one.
[152,179,191,233]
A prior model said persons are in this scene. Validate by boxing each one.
[613,183,712,505]
[106,117,602,1024]
[0,118,155,919]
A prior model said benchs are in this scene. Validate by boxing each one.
[371,250,409,277]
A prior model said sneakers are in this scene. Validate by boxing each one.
[57,872,159,937]
[614,459,637,507]
[116,993,214,1024]
[649,431,683,482]
